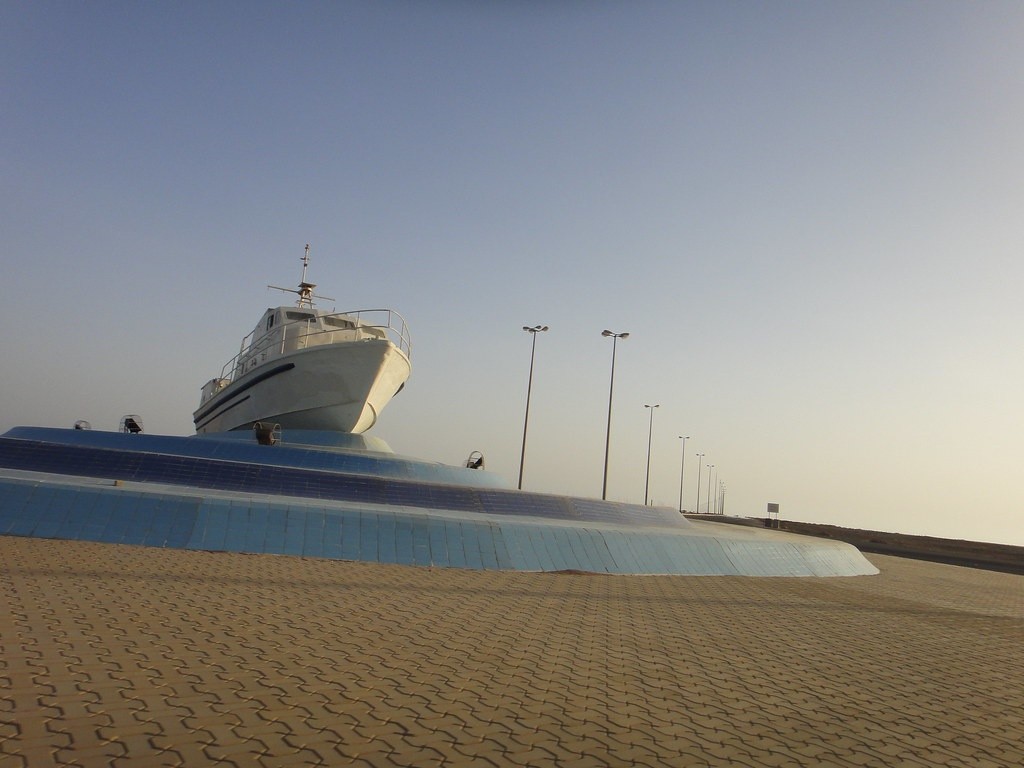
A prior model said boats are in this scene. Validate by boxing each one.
[196,244,412,437]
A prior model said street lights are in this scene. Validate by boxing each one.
[600,330,630,500]
[645,404,659,507]
[678,436,690,513]
[706,464,714,513]
[518,325,550,489]
[695,453,705,513]
